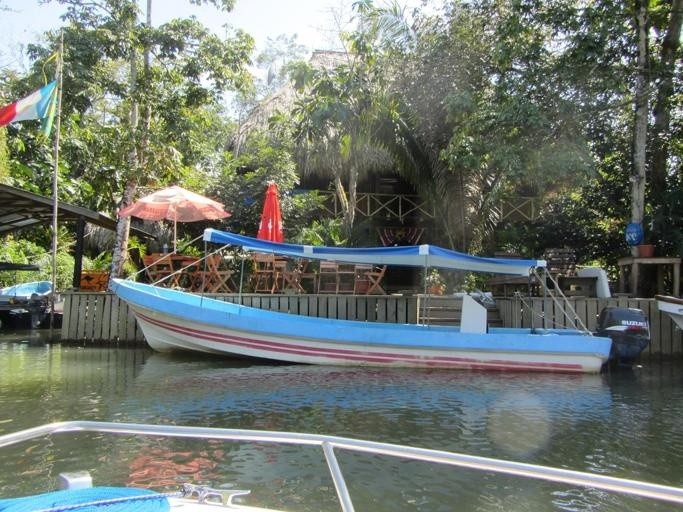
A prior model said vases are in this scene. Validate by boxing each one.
[638,245,653,258]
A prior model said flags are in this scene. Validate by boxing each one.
[0,80,60,125]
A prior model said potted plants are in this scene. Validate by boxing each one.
[426,268,447,295]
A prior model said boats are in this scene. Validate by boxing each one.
[0,422,682,512]
[655,295,683,330]
[107,227,651,373]
[0,262,55,329]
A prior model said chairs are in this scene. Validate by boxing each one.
[140,250,387,295]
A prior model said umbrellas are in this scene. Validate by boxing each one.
[117,181,227,255]
[254,183,284,242]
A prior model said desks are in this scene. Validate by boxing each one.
[618,256,681,298]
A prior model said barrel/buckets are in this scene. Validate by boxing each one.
[544,247,577,276]
